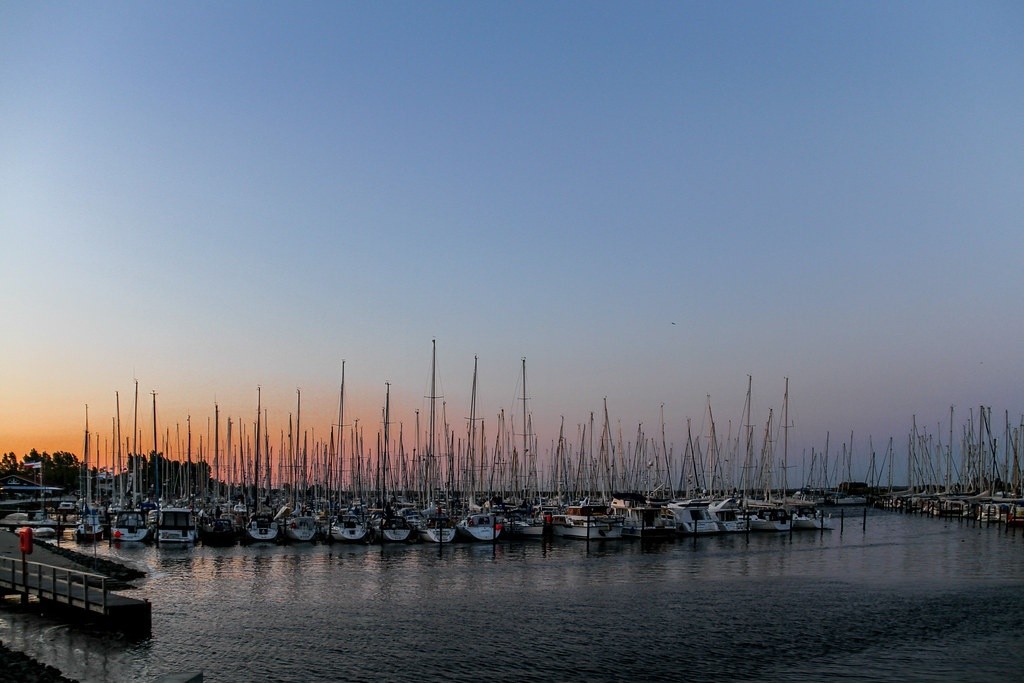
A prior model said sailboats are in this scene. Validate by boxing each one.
[0,336,1023,549]
